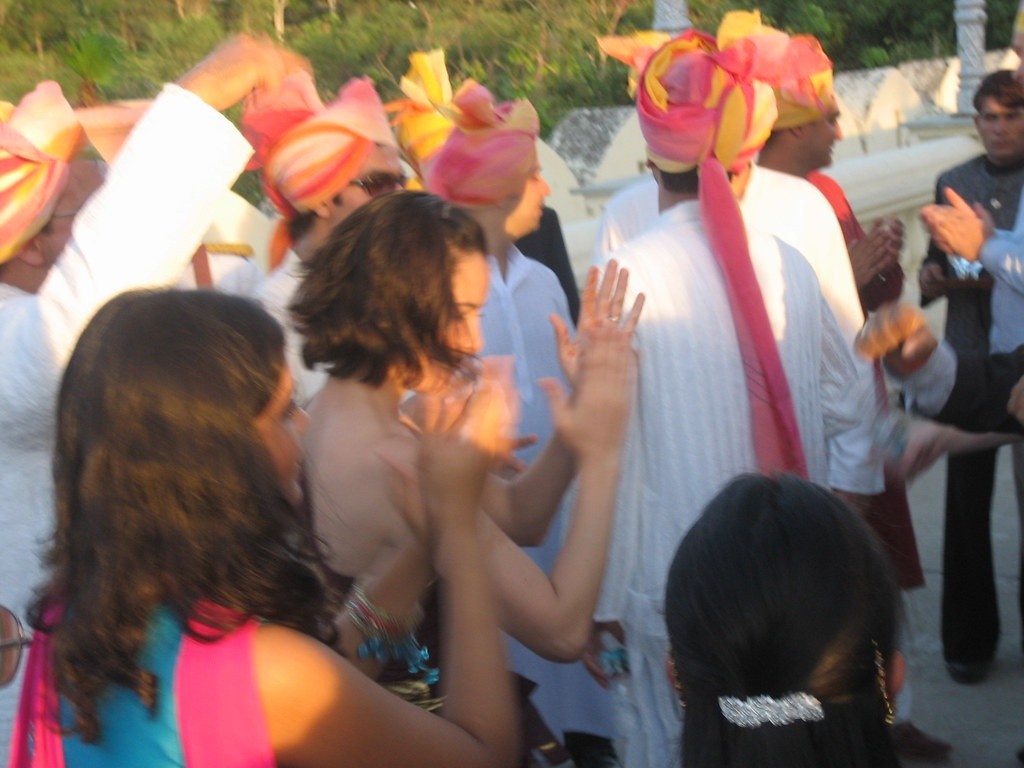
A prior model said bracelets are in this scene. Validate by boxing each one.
[347,578,425,646]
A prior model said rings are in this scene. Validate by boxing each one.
[609,317,620,322]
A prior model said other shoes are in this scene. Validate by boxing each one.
[943,645,989,681]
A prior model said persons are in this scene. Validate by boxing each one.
[0,31,284,768]
[83,0,1024,768]
[662,472,953,768]
[11,291,520,768]
[286,190,646,767]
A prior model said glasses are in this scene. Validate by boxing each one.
[0,604,33,686]
[351,170,407,198]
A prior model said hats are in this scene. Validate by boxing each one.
[593,26,811,480]
[382,48,540,211]
[0,80,81,267]
[715,9,837,132]
[239,50,399,270]
